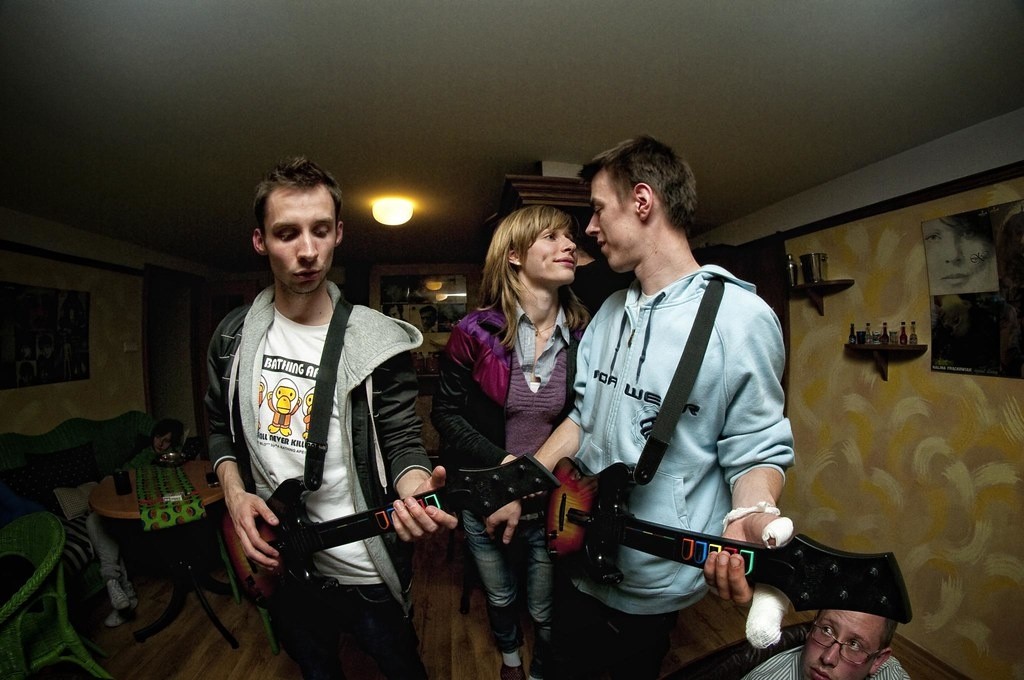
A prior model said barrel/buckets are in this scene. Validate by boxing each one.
[799,253,828,283]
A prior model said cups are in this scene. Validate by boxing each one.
[856,331,866,344]
[113,471,132,495]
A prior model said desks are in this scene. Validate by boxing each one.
[88,448,240,649]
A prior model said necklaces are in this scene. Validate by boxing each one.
[533,322,555,336]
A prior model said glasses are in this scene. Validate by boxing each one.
[810,614,882,666]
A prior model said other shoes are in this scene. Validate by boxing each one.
[527,656,543,680]
[500,660,526,679]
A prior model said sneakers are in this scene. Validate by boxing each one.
[107,578,131,611]
[104,596,139,628]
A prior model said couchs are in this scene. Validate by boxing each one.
[0,409,157,608]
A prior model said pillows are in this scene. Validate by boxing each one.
[0,441,100,529]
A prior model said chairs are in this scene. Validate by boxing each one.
[661,621,814,680]
[0,509,115,680]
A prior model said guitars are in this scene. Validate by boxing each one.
[541,456,914,625]
[218,450,562,614]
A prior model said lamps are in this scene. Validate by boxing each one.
[372,198,416,226]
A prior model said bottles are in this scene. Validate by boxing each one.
[890,332,898,344]
[909,321,918,345]
[412,350,440,375]
[873,331,880,343]
[849,323,856,344]
[880,323,889,343]
[899,322,908,344]
[866,323,871,343]
[786,255,797,287]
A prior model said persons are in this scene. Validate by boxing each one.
[201,155,457,680]
[740,609,913,680]
[85,419,185,626]
[428,205,591,680]
[483,134,795,680]
[420,306,438,331]
[921,210,995,294]
[381,304,408,321]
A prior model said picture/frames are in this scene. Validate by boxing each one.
[0,280,90,391]
